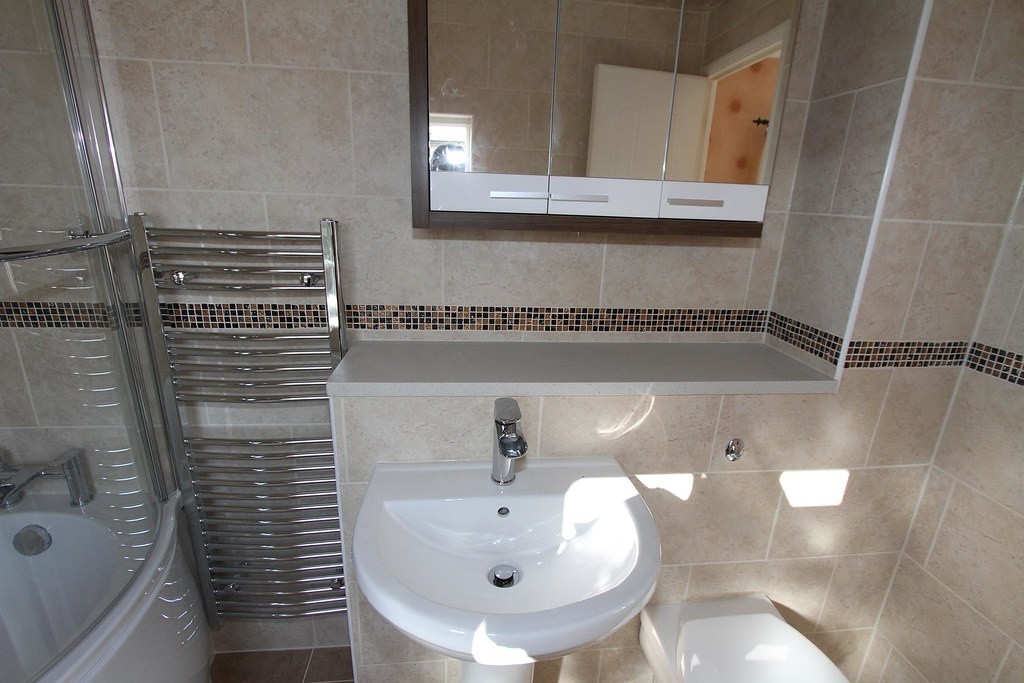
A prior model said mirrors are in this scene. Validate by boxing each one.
[427,0,558,177]
[550,0,684,182]
[664,0,802,186]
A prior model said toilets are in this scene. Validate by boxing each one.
[637,592,853,683]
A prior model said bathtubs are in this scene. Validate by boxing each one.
[0,499,216,683]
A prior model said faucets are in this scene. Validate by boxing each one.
[492,396,529,486]
[51,447,95,508]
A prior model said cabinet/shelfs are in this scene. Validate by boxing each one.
[407,0,803,239]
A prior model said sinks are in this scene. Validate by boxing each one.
[353,470,665,669]
